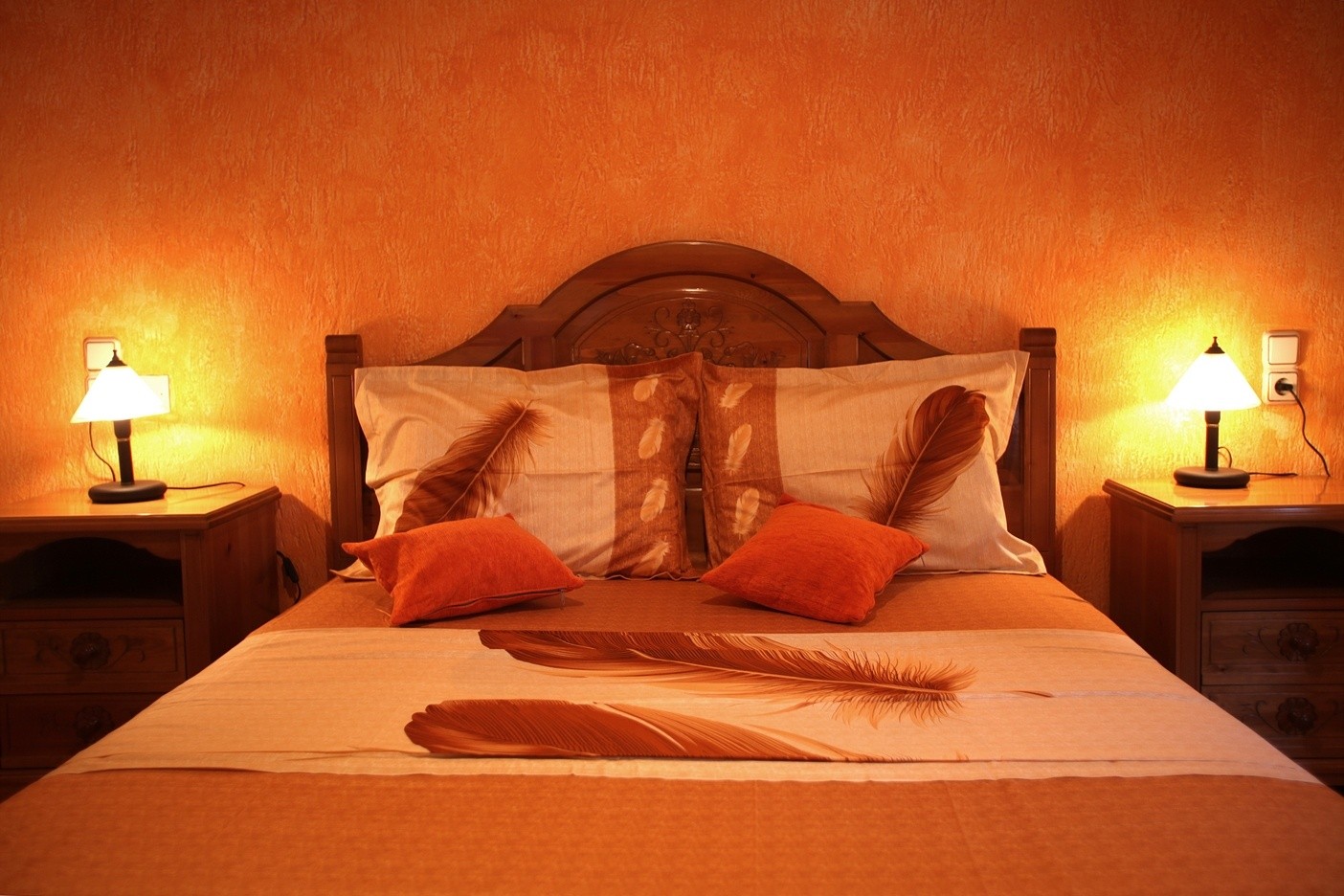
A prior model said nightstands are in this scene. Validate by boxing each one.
[1103,478,1344,790]
[0,482,281,802]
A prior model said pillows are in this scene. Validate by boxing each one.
[330,351,704,582]
[695,348,1047,576]
[702,493,928,626]
[339,511,586,628]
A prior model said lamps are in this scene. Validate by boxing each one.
[1165,335,1261,489]
[70,347,169,505]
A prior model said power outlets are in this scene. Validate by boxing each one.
[1261,367,1299,405]
[86,375,98,391]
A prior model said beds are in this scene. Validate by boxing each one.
[1,240,1344,895]
